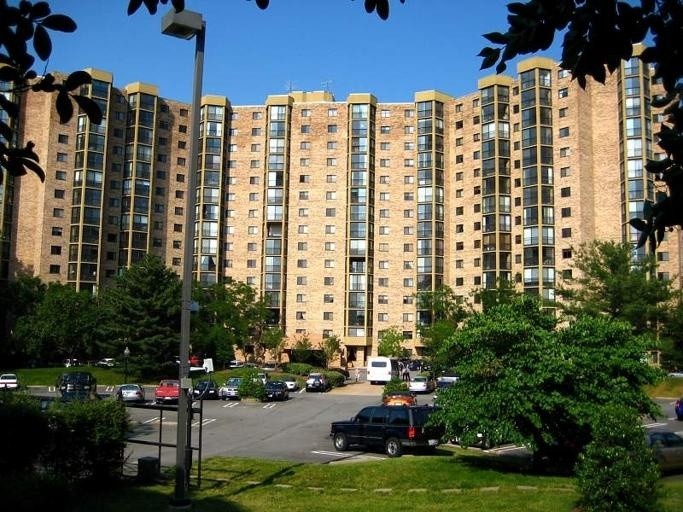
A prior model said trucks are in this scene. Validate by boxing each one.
[366,355,400,385]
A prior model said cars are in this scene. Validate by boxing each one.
[62,358,80,369]
[250,373,271,386]
[192,378,220,401]
[330,367,350,380]
[56,374,72,389]
[229,358,285,373]
[118,384,145,404]
[640,430,682,477]
[674,397,683,421]
[407,374,435,395]
[170,353,200,368]
[0,372,19,392]
[448,428,514,449]
[403,360,431,372]
[281,376,297,392]
[260,380,289,402]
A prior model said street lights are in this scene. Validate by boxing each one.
[123,346,131,383]
[160,5,207,510]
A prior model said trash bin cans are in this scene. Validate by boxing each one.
[137,457,161,480]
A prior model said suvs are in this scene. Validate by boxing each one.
[304,372,331,393]
[96,359,116,369]
[60,372,96,398]
[328,403,444,459]
[218,377,246,400]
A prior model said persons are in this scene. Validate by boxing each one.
[354,367,362,383]
[399,362,403,375]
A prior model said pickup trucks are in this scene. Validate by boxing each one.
[436,371,460,388]
[155,379,180,404]
